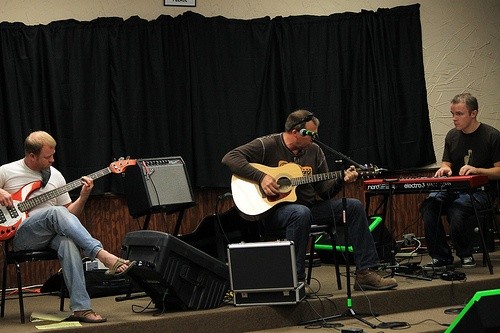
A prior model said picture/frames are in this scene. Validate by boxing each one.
[164,0,196,7]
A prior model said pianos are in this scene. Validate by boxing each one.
[360,169,494,276]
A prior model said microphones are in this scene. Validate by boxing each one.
[300,129,316,136]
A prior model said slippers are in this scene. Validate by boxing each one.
[105,257,137,278]
[69,309,107,323]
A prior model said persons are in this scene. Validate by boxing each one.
[422,93,500,269]
[0,132,136,323]
[222,111,398,293]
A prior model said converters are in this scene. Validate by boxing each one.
[341,328,363,333]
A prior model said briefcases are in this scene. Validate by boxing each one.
[227,239,307,306]
[85,268,140,299]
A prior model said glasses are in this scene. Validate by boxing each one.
[288,112,314,131]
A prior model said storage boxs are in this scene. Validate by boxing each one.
[226,240,306,307]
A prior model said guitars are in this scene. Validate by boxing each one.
[229,161,379,222]
[0,155,136,243]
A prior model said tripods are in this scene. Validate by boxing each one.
[297,140,379,328]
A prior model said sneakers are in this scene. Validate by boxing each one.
[421,256,455,269]
[460,254,476,267]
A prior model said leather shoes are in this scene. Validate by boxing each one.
[354,266,399,290]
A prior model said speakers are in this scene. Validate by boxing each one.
[442,288,500,333]
[119,231,230,310]
[314,215,397,266]
[122,156,196,220]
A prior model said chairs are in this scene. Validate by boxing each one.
[258,218,341,290]
[1,241,85,324]
